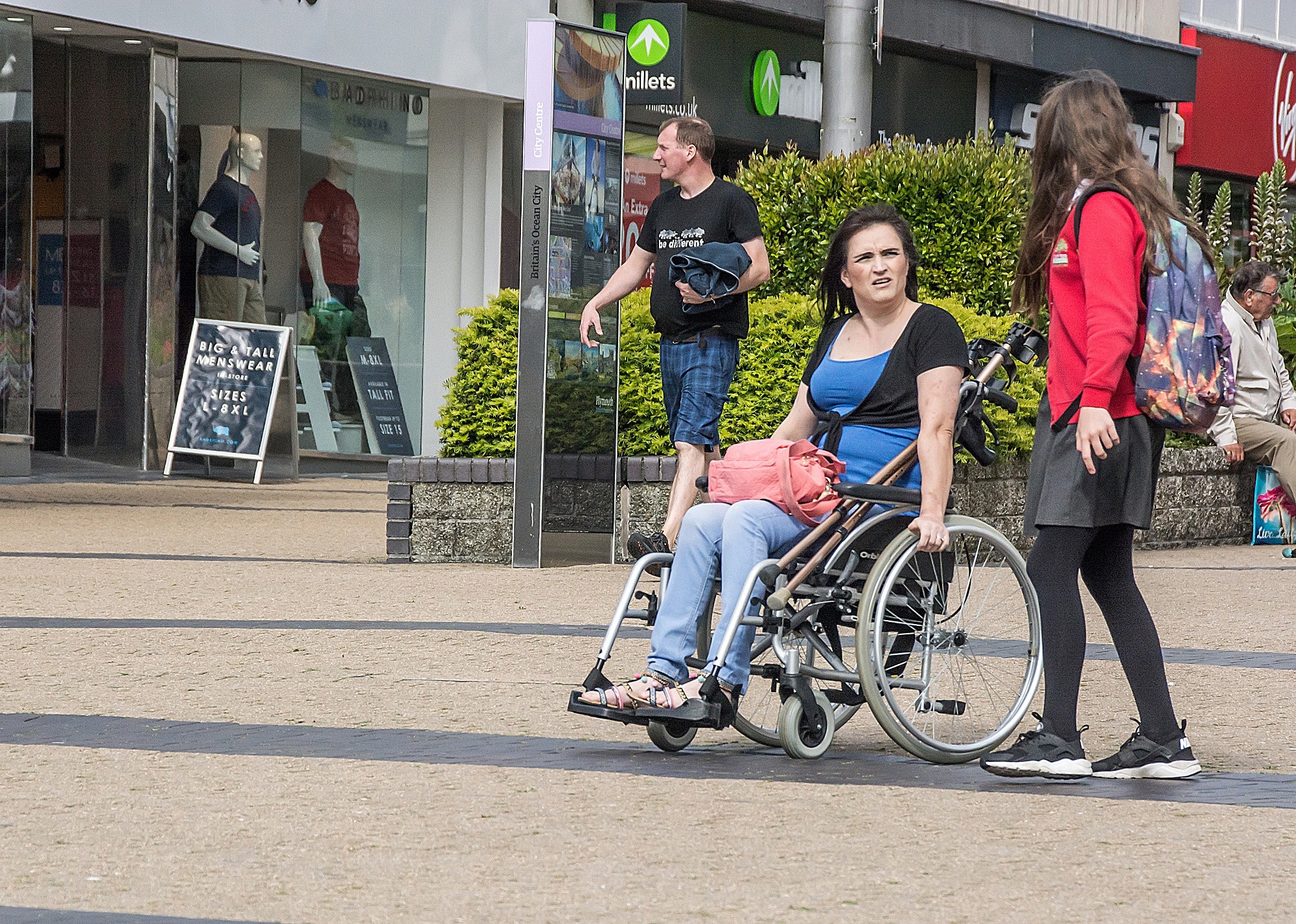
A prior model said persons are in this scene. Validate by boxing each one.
[979,68,1203,780]
[190,131,268,324]
[579,115,771,575]
[578,202,971,713]
[589,138,600,216]
[1205,261,1296,509]
[298,139,371,422]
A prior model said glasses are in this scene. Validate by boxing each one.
[1244,287,1280,299]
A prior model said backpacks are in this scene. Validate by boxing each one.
[1074,181,1236,437]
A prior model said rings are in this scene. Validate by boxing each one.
[937,537,944,542]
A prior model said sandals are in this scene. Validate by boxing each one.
[577,667,677,710]
[626,673,736,710]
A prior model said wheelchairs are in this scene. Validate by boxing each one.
[566,324,1050,763]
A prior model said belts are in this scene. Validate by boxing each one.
[672,335,698,344]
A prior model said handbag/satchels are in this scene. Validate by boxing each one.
[707,437,848,528]
[1251,465,1296,546]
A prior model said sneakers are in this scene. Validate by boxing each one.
[1093,717,1203,779]
[626,532,677,578]
[980,712,1094,780]
